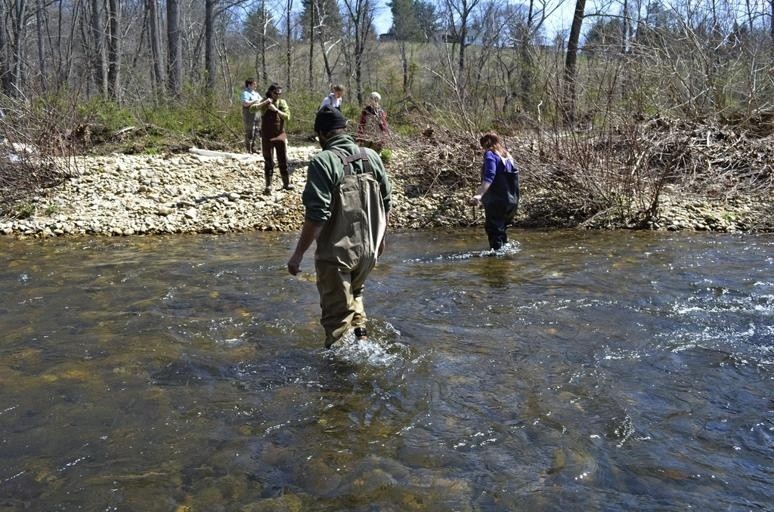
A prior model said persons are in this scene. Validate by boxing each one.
[241,77,266,154]
[287,101,392,348]
[248,82,296,192]
[320,83,346,113]
[465,132,521,254]
[357,91,392,154]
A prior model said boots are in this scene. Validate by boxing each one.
[281,171,295,191]
[265,174,272,192]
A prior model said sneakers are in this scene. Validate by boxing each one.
[354,328,367,339]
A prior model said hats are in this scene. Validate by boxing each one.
[314,104,346,130]
